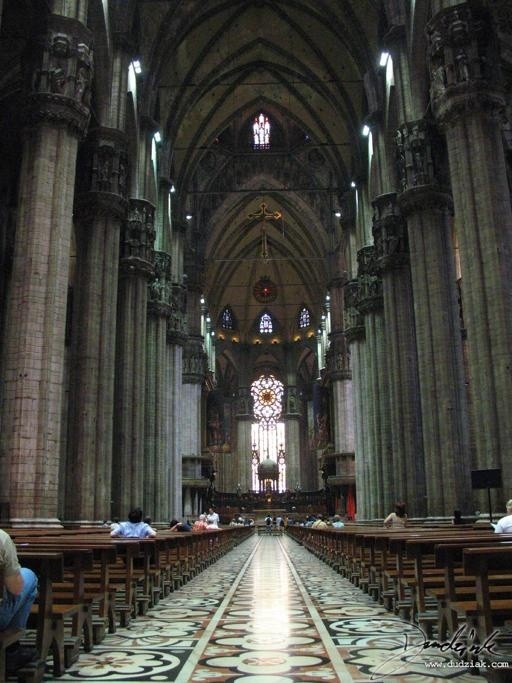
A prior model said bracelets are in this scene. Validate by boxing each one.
[175,523,179,528]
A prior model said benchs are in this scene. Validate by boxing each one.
[283,519,512,683]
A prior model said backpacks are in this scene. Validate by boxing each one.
[267,517,270,525]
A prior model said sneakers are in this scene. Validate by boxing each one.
[5,647,32,671]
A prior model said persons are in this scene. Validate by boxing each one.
[144,515,157,531]
[452,510,465,524]
[110,516,123,530]
[384,498,408,528]
[102,519,110,528]
[209,519,219,543]
[490,498,512,543]
[0,529,40,672]
[112,507,156,568]
[208,507,220,526]
[169,519,191,531]
[265,512,343,535]
[195,518,207,529]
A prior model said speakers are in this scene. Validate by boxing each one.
[471,468,502,489]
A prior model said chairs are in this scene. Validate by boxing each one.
[0,519,255,683]
